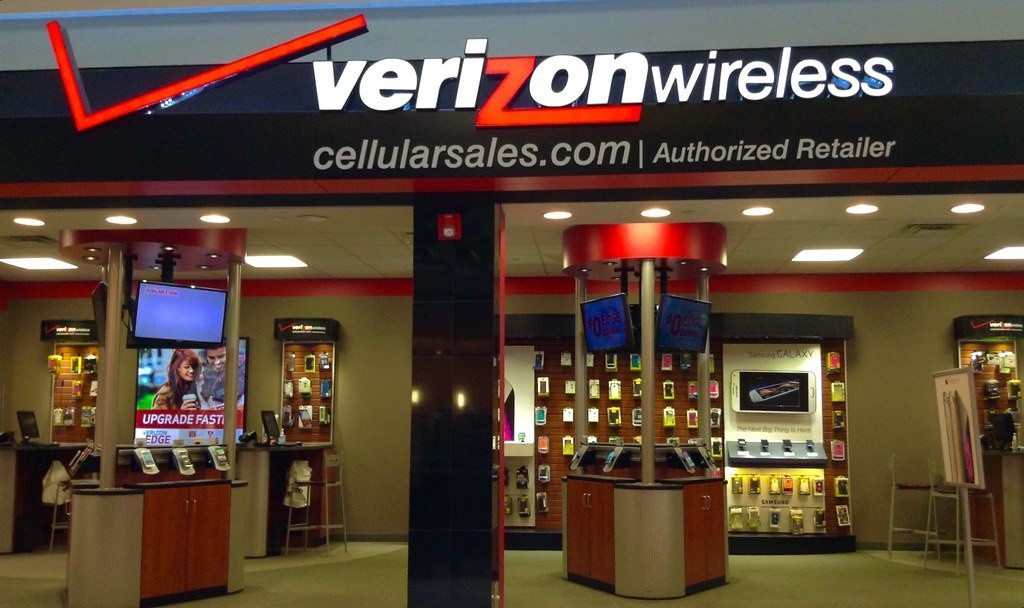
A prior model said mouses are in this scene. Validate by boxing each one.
[295,441,302,445]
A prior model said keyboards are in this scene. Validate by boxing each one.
[277,443,298,446]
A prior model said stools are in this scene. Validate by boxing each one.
[285,451,349,558]
[887,452,1001,576]
[48,440,100,552]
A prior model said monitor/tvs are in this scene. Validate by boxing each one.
[17,411,40,444]
[580,292,712,354]
[127,279,228,350]
[261,410,280,443]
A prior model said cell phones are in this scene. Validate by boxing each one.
[606,449,616,463]
[178,451,193,467]
[682,450,695,468]
[141,451,155,467]
[807,440,813,446]
[216,448,228,464]
[739,439,746,445]
[706,449,715,464]
[762,440,768,445]
[730,369,816,414]
[783,440,791,446]
